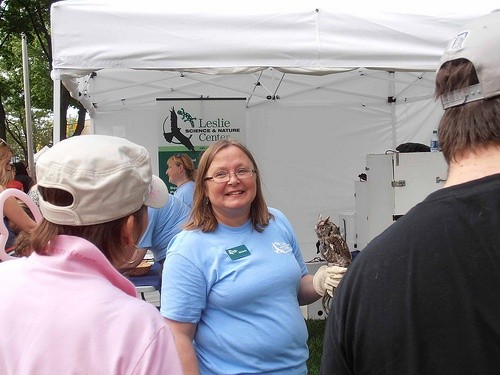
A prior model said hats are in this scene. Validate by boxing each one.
[436,10,500,109]
[36,135,168,226]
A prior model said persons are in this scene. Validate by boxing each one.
[0,135,186,375]
[159,139,348,375]
[322,5,500,375]
[0,138,38,266]
[165,152,197,210]
[119,192,195,270]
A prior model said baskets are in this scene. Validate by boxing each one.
[126,261,152,276]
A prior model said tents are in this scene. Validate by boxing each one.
[49,0,500,262]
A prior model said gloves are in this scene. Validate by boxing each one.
[313,265,347,298]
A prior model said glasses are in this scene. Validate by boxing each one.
[201,169,257,182]
[0,139,7,145]
[176,155,186,166]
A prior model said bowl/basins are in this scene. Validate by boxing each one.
[126,262,152,276]
